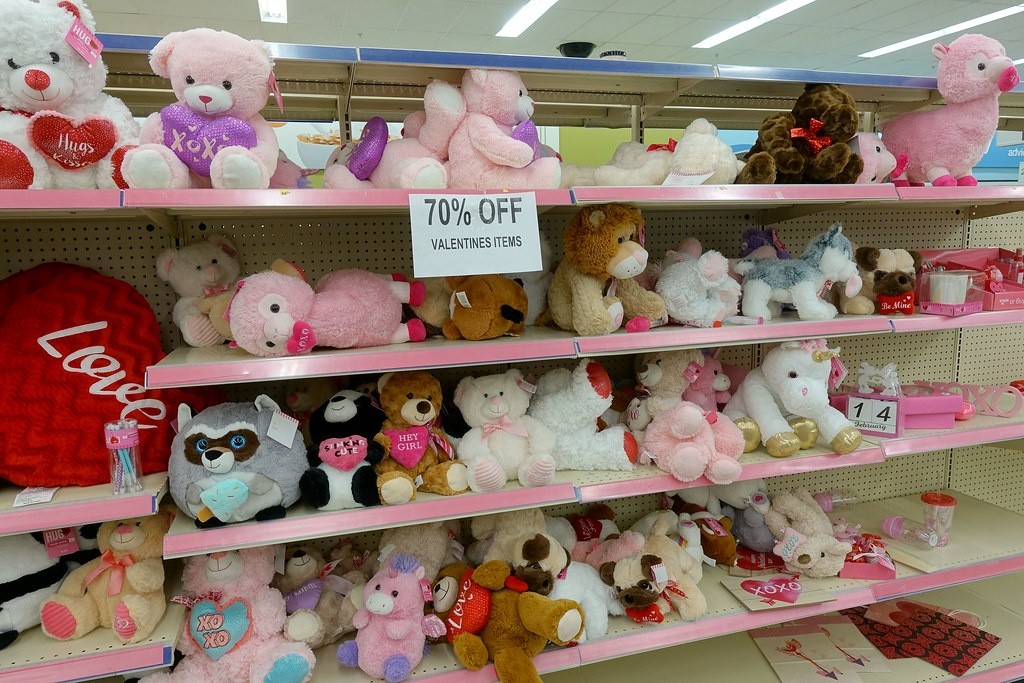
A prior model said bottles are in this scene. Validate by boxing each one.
[813,489,857,513]
[878,515,939,549]
[1008,248,1024,284]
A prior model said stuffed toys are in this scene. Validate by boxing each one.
[547,201,668,337]
[851,132,897,184]
[269,147,321,188]
[39,503,179,642]
[137,543,316,683]
[647,249,742,328]
[448,68,563,189]
[0,524,70,650]
[156,233,555,356]
[733,222,862,320]
[120,28,280,190]
[593,117,747,186]
[880,34,1020,186]
[0,0,139,190]
[736,84,864,185]
[633,237,776,291]
[626,338,863,485]
[543,504,707,624]
[64,523,102,572]
[662,479,852,579]
[269,507,627,683]
[323,78,467,189]
[737,224,796,310]
[821,246,922,315]
[167,361,639,529]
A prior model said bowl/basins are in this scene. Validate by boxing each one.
[600,50,626,60]
[555,42,596,58]
[297,140,340,170]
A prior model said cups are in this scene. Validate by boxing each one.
[103,419,145,495]
[920,493,957,548]
[929,273,973,306]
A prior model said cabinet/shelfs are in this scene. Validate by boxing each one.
[573,173,907,683]
[122,184,583,683]
[757,181,1024,683]
[0,186,189,683]
